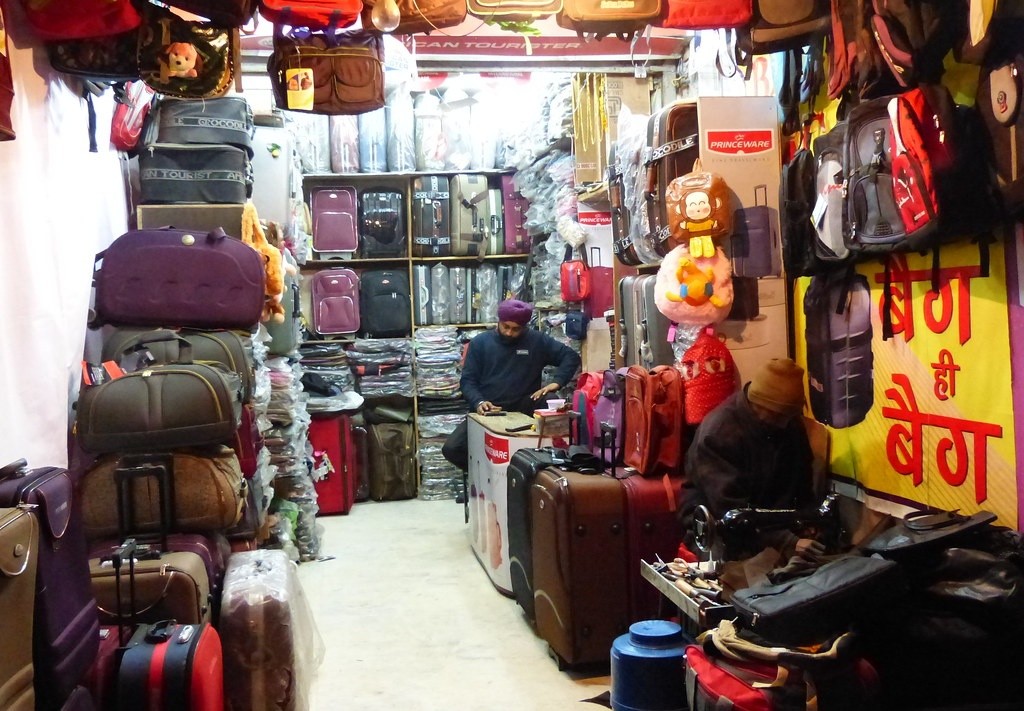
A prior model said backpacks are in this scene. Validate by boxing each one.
[780,0,1024,315]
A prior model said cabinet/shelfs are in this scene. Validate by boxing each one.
[294,169,531,499]
[577,95,791,391]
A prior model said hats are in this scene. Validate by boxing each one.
[499,300,533,326]
[747,355,806,418]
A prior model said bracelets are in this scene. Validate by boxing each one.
[476,401,484,407]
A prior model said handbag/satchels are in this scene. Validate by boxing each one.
[267,29,386,114]
[85,225,265,331]
[369,421,416,501]
[0,0,828,208]
[685,508,1024,711]
[80,327,256,536]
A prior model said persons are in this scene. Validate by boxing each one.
[440,300,582,504]
[673,357,827,563]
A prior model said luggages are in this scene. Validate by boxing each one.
[263,274,301,354]
[359,268,411,340]
[728,184,781,280]
[221,549,297,711]
[803,272,875,429]
[308,410,355,514]
[310,182,358,260]
[0,458,223,711]
[359,186,405,259]
[310,265,361,340]
[506,209,733,673]
[410,174,534,325]
[589,247,613,320]
